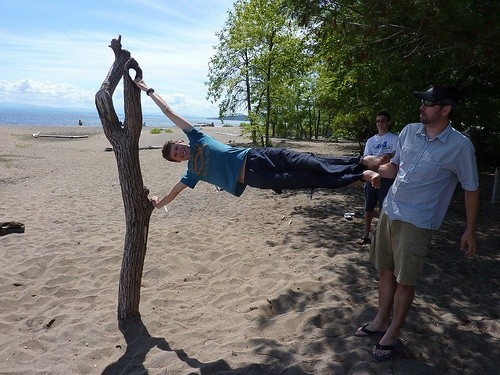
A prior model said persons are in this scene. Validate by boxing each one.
[132,76,395,209]
[347,83,480,361]
[358,112,400,244]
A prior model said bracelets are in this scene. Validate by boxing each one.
[147,87,154,96]
